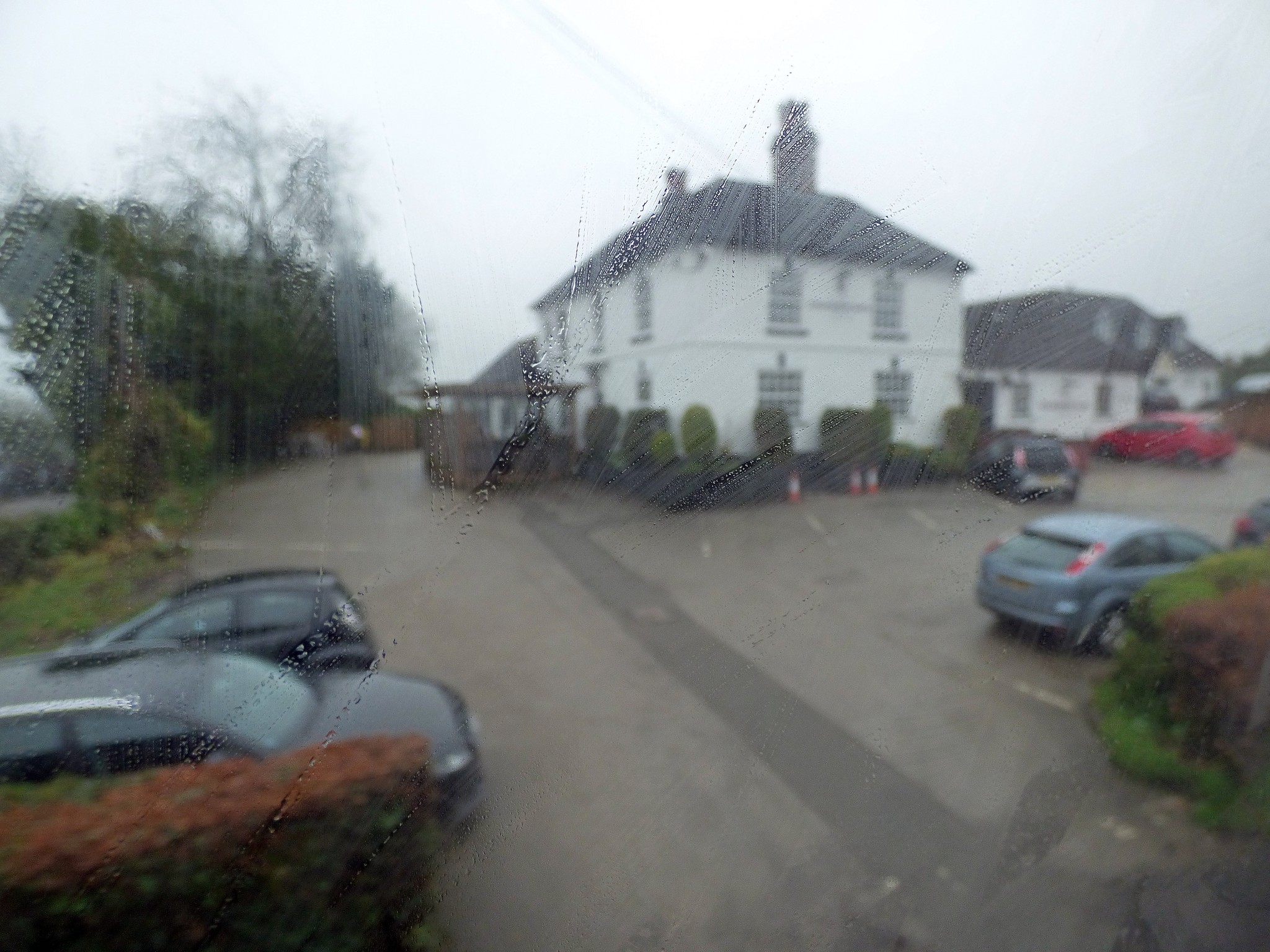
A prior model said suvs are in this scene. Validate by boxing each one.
[1,643,486,824]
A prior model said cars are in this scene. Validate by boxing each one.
[974,516,1227,657]
[41,565,380,676]
[1090,412,1236,470]
[1235,501,1269,549]
[973,434,1080,501]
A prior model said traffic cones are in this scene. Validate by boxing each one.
[865,466,879,495]
[848,467,861,496]
[788,471,802,505]
